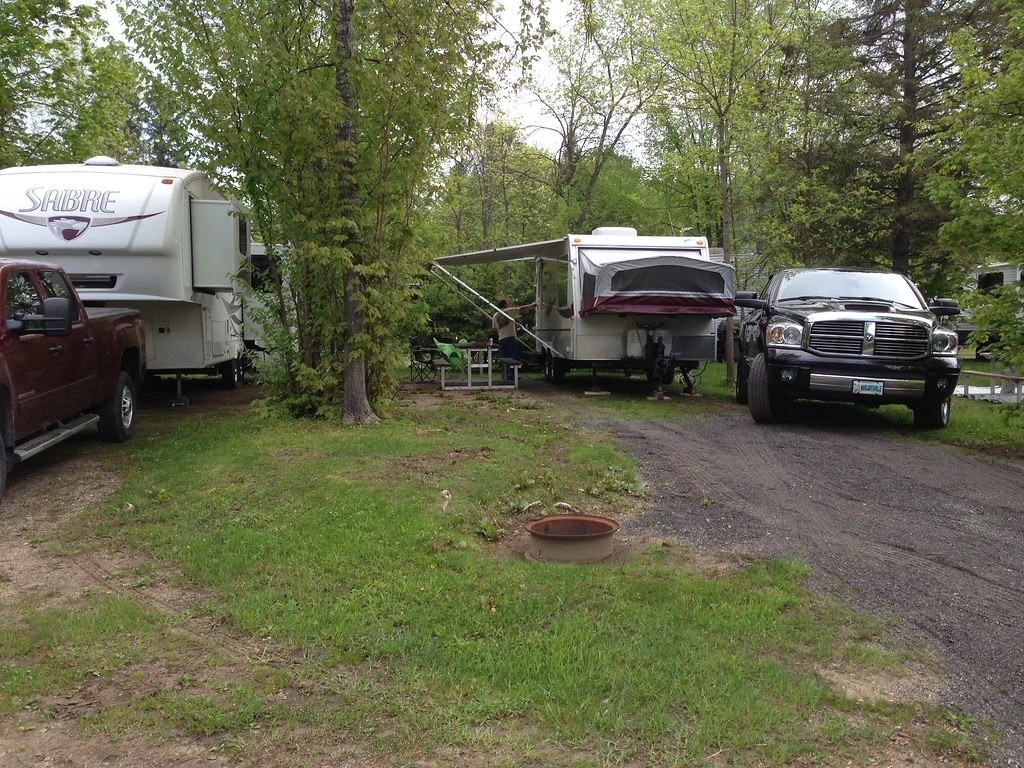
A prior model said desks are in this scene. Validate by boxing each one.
[445,341,515,397]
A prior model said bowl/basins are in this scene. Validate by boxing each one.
[523,514,620,563]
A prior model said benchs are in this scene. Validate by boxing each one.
[496,358,523,389]
[433,359,450,391]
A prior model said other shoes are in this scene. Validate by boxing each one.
[507,376,521,380]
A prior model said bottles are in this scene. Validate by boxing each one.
[489,337,493,345]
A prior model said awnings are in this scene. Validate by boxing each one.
[581,254,737,317]
[426,239,567,357]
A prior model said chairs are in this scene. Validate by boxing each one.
[409,336,438,383]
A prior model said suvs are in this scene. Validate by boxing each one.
[734,266,962,431]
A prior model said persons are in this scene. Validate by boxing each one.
[492,300,537,379]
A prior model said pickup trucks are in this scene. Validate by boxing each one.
[0,257,147,501]
